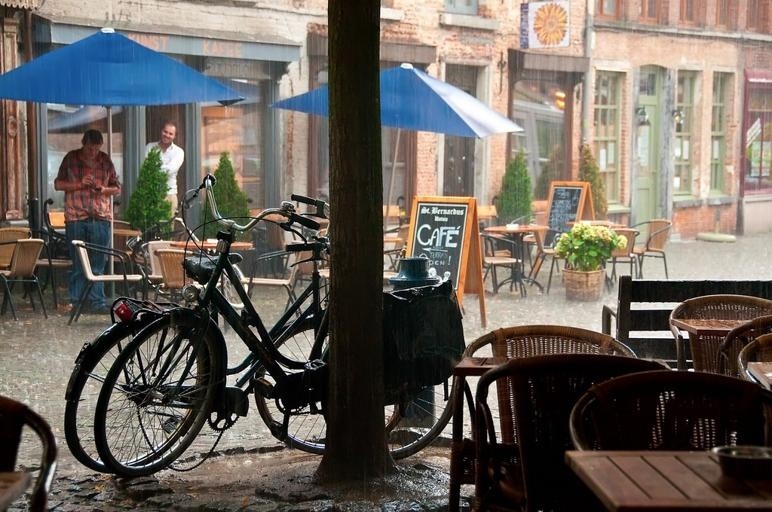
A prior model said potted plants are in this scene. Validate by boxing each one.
[495,148,534,259]
[579,141,609,267]
[200,150,252,242]
[127,147,171,293]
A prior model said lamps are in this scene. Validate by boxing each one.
[635,105,651,135]
[671,110,683,123]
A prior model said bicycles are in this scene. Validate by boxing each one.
[60,173,464,480]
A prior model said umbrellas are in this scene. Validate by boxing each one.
[1,26,248,303]
[48,79,263,135]
[267,60,526,232]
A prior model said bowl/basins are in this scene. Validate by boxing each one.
[709,444,770,476]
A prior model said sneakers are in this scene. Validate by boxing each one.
[64,305,88,317]
[98,306,110,314]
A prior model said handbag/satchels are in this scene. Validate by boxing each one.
[384,280,465,390]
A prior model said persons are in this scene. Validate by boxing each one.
[144,120,186,240]
[53,127,121,313]
